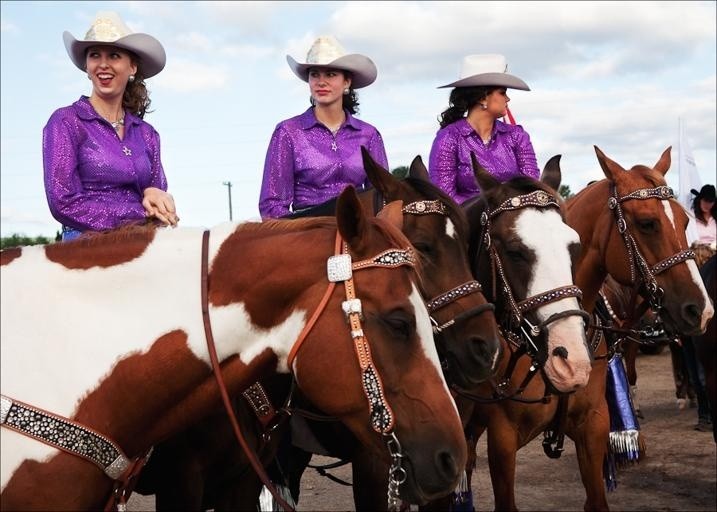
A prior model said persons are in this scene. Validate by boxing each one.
[255,33,389,226]
[687,183,716,253]
[38,10,181,247]
[426,53,539,208]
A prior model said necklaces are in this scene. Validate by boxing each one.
[323,121,344,134]
[479,134,492,147]
[105,118,126,131]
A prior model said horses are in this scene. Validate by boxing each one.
[285,145,594,512]
[275,147,501,393]
[468,144,715,511]
[0,187,469,512]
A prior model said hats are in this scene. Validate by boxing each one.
[691,184,717,202]
[436,54,530,92]
[286,34,377,90]
[62,15,166,79]
